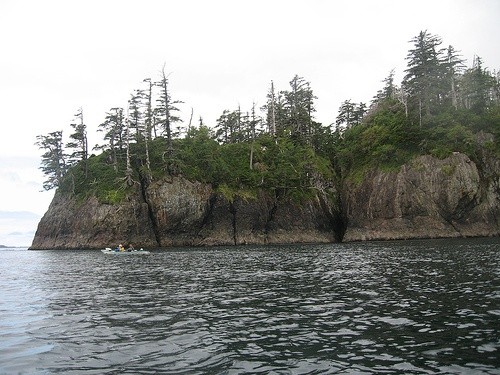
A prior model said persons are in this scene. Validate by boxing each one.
[117,244,124,252]
[127,244,134,252]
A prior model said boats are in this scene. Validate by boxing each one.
[100,247,151,256]
